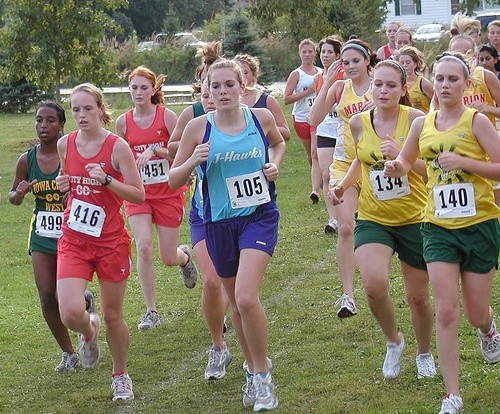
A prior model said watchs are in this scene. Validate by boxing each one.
[150,145,157,154]
[101,174,113,186]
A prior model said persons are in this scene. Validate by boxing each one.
[169,43,286,412]
[115,66,199,329]
[397,46,433,114]
[313,38,338,232]
[469,20,500,79]
[388,29,428,78]
[282,38,322,203]
[235,52,291,141]
[55,84,145,399]
[309,39,377,318]
[162,63,231,380]
[377,21,400,61]
[9,102,95,372]
[450,13,500,207]
[384,52,500,414]
[327,60,434,379]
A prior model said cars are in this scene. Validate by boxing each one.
[138,41,152,53]
[412,24,446,43]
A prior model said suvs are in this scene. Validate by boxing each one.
[153,32,205,53]
[475,13,500,30]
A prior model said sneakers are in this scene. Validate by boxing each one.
[110,374,134,400]
[324,221,337,234]
[178,245,199,289]
[83,290,95,313]
[78,313,100,369]
[415,353,436,379]
[335,293,357,318]
[382,332,404,380]
[477,319,500,366]
[240,359,257,407]
[438,392,463,414]
[55,351,79,373]
[138,310,160,330]
[204,343,231,380]
[310,192,319,206]
[253,357,278,412]
